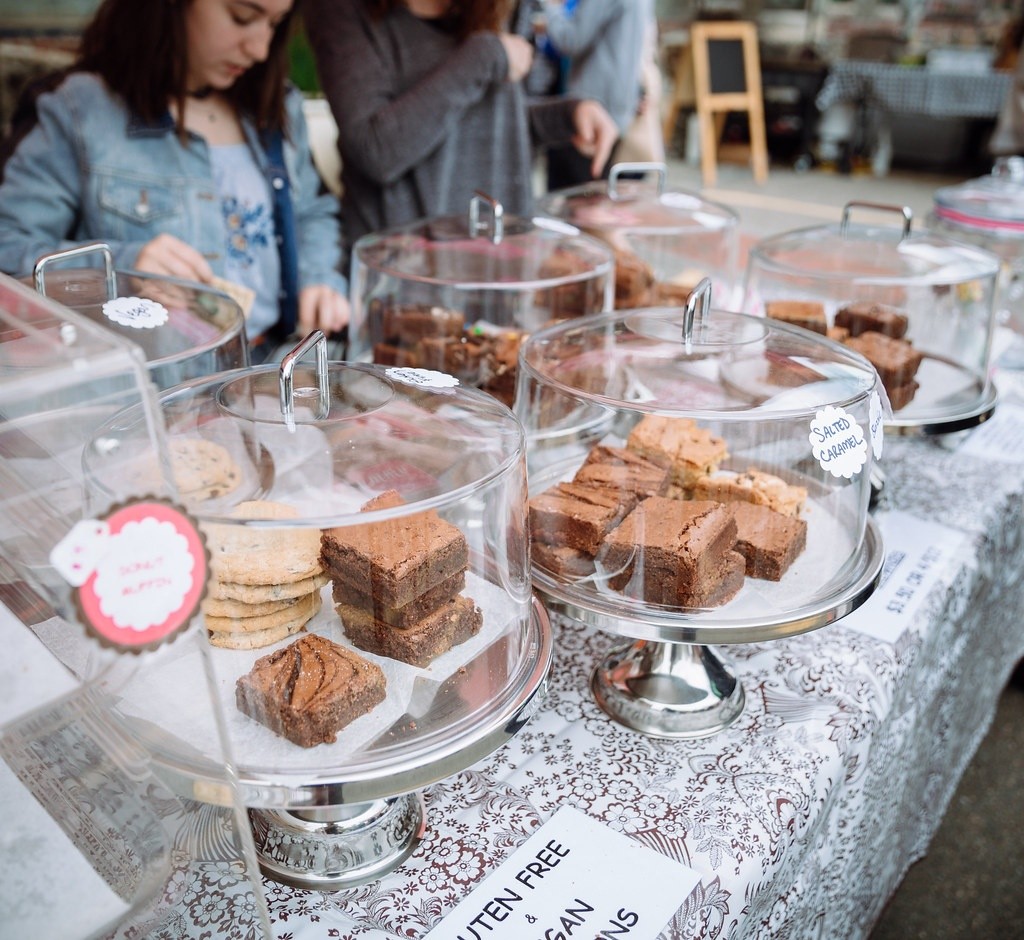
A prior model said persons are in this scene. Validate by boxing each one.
[510,2,653,192]
[0,0,353,380]
[294,0,622,260]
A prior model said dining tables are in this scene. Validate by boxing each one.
[0,160,1023,940]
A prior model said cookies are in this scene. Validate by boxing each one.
[138,436,333,653]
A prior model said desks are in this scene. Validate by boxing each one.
[815,60,1018,176]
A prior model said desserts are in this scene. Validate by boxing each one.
[368,248,925,613]
[236,634,389,747]
[319,488,486,669]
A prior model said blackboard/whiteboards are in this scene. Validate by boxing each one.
[673,20,763,108]
